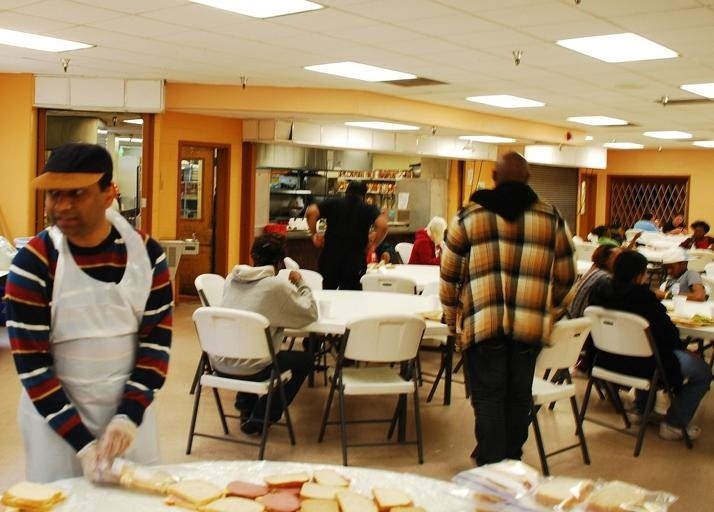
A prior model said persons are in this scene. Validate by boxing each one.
[564,244,623,373]
[589,249,713,442]
[407,216,448,266]
[208,231,318,436]
[591,213,714,302]
[306,181,389,291]
[5,144,175,486]
[439,151,578,468]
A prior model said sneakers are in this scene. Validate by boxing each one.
[240,409,275,434]
[630,408,667,424]
[659,422,701,441]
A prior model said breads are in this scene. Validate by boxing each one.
[165,467,423,512]
[1,481,67,511]
[475,460,650,512]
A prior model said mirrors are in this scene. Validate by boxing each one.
[37,110,149,236]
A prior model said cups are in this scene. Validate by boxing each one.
[672,294,688,312]
[398,192,409,209]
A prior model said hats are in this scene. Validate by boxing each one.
[31,144,112,190]
[662,247,700,264]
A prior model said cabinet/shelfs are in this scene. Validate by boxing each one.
[339,175,399,212]
